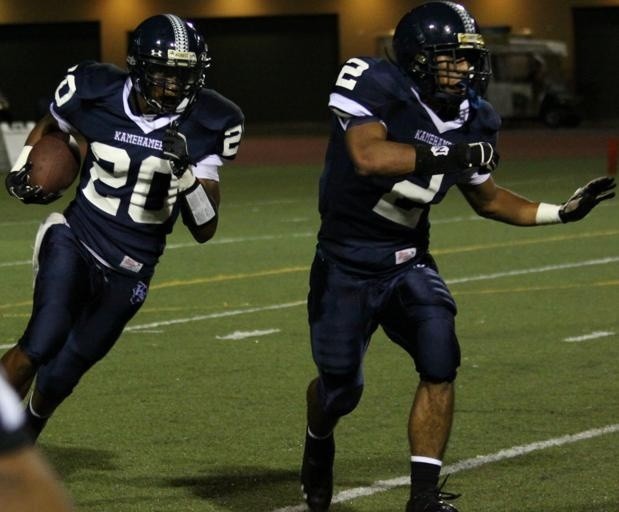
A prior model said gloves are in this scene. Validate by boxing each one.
[464,141,500,175]
[559,176,616,224]
[162,121,192,176]
[7,162,63,205]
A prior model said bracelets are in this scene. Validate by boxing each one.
[415,141,470,175]
[10,145,33,171]
[534,198,567,226]
[174,168,217,236]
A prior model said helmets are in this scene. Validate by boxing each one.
[128,14,208,115]
[392,0,485,101]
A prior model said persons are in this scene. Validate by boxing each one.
[298,0,619,511]
[1,11,245,445]
[0,363,89,510]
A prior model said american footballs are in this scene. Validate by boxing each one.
[27,129,80,193]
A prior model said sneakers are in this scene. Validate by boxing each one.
[298,416,337,512]
